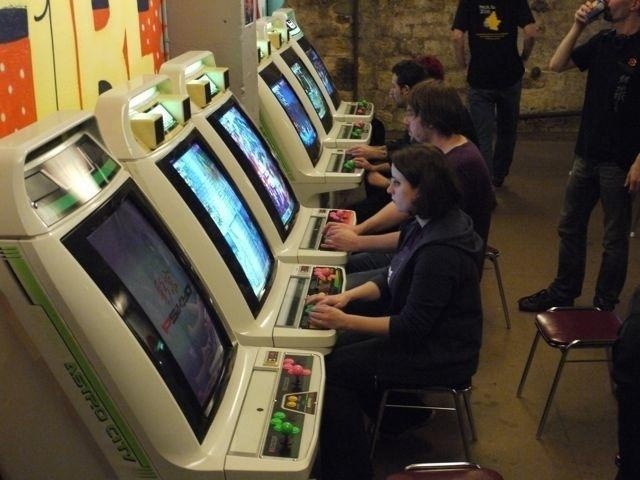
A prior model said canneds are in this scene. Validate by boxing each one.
[579,0,605,24]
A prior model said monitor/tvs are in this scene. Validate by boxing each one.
[280,46,333,135]
[258,61,323,168]
[207,95,300,244]
[155,126,274,320]
[60,176,234,444]
[296,35,340,111]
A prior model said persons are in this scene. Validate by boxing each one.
[453,1,536,187]
[303,143,485,480]
[611,285,640,478]
[319,78,497,290]
[345,57,481,225]
[516,0,640,309]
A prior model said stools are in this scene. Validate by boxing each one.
[368,383,477,460]
[515,307,624,440]
[386,461,504,480]
[484,245,512,330]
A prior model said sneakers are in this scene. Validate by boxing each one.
[366,394,436,440]
[519,284,575,312]
[494,171,507,192]
